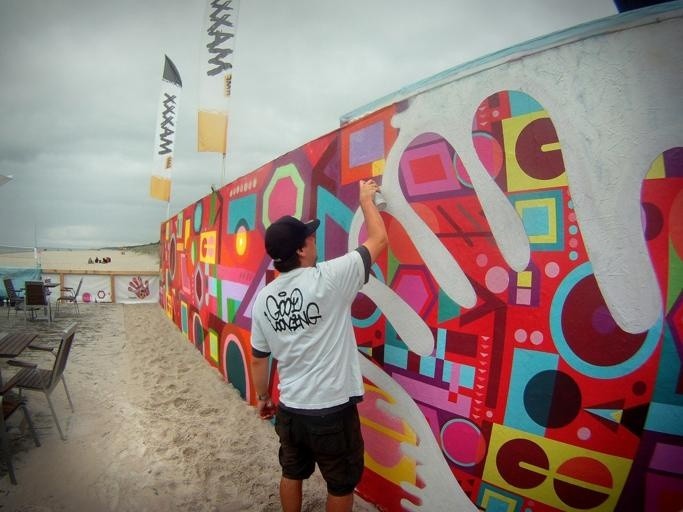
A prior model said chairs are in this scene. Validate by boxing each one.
[0,277,85,485]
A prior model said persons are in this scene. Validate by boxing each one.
[245,176,394,511]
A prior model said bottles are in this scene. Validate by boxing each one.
[373,190,386,210]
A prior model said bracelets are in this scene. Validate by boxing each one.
[254,392,271,403]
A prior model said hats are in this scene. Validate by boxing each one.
[262,215,320,259]
